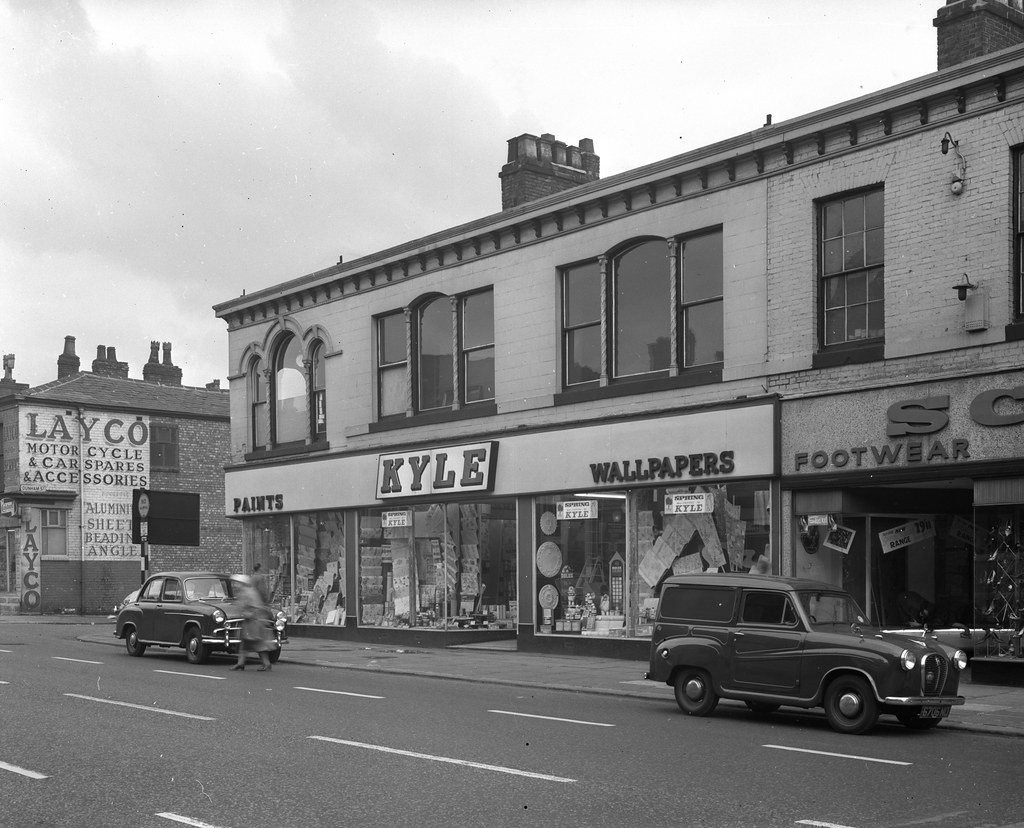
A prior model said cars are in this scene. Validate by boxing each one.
[113,572,289,665]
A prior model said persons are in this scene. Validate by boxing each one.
[229,574,277,672]
[250,563,268,606]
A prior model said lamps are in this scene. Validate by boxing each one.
[951,272,978,301]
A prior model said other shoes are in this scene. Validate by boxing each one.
[230,664,245,672]
[257,665,272,671]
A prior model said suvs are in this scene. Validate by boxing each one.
[645,572,967,734]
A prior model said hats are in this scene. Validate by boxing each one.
[230,574,251,585]
